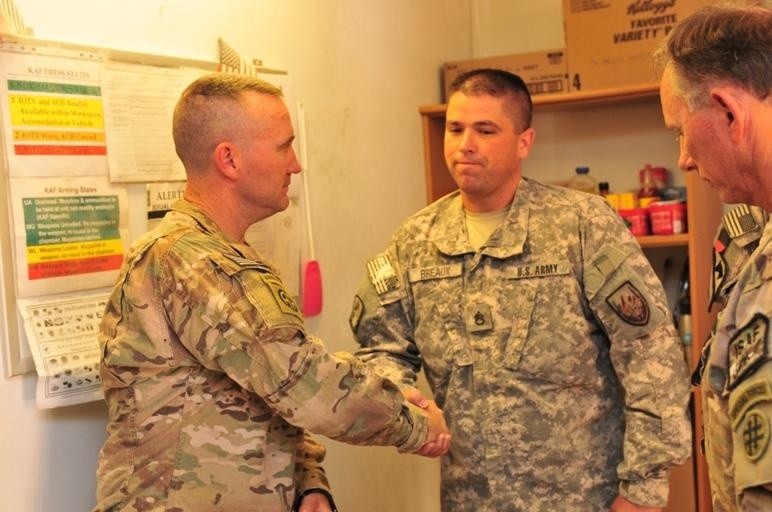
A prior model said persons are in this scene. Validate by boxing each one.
[651,4,772,511]
[350,70,694,512]
[92,72,449,512]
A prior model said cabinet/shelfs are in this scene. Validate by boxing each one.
[418,85,725,512]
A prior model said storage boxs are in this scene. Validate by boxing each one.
[666,447,697,512]
[443,46,568,105]
[562,0,760,92]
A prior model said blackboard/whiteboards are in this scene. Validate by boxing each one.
[0,0,301,376]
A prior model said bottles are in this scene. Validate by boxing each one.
[567,166,597,194]
[677,259,692,345]
[638,170,661,210]
[598,183,609,197]
